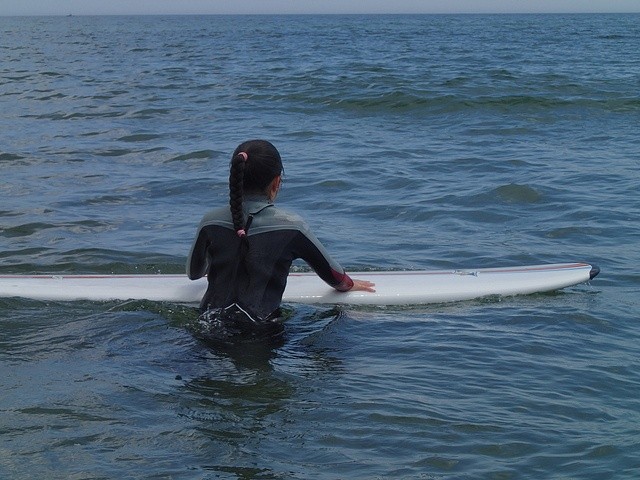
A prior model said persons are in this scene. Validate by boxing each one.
[186,139,376,345]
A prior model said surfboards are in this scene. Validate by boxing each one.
[0,261,600,304]
